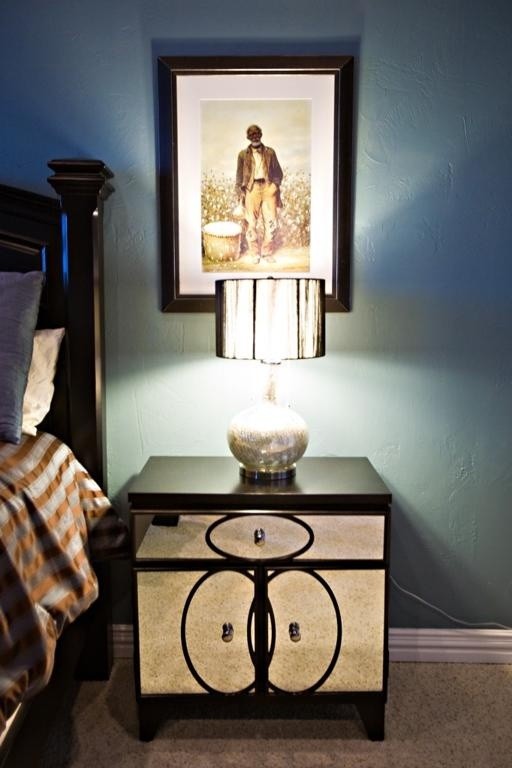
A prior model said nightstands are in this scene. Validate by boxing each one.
[129,454,395,743]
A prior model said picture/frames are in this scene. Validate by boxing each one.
[155,51,357,316]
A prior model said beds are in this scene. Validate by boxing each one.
[0,157,116,766]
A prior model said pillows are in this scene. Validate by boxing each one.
[0,266,66,444]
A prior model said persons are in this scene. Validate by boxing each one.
[235,124,285,266]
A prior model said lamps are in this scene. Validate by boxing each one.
[212,279,325,481]
